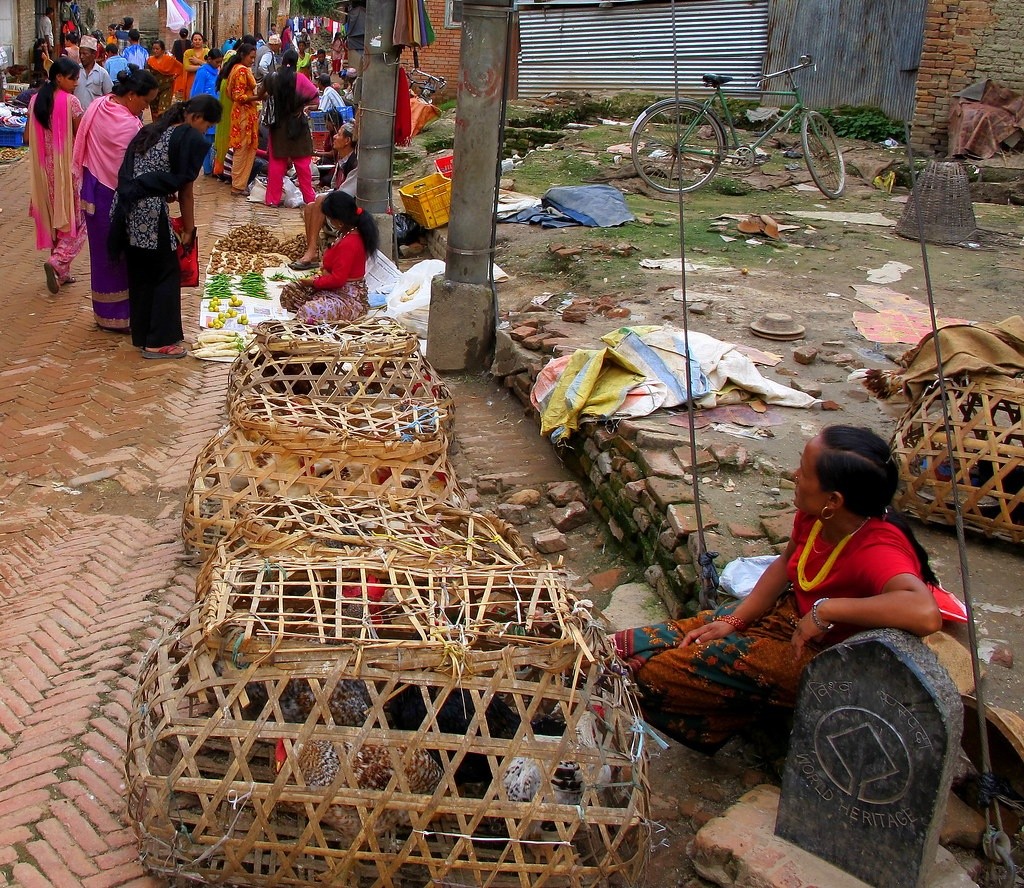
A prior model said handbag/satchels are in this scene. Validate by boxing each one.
[260,71,277,128]
[393,211,421,245]
[286,115,309,139]
[164,198,199,287]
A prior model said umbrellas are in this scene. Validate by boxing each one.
[392,0,437,69]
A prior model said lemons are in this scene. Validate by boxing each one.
[208,295,249,329]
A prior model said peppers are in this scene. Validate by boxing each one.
[267,269,317,282]
[202,273,267,299]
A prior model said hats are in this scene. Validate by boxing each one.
[346,68,357,78]
[315,72,331,83]
[343,118,356,134]
[79,35,98,51]
[268,34,282,44]
[302,28,306,33]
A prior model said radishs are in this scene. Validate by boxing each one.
[190,329,241,357]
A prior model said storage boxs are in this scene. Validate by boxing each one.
[310,105,353,150]
[398,173,452,229]
[434,154,453,179]
[0,117,28,148]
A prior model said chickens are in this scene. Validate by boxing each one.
[864,345,922,402]
[391,678,567,820]
[235,572,385,727]
[275,739,445,881]
[245,350,464,552]
[498,701,612,864]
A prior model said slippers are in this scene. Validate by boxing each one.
[135,345,144,352]
[141,343,187,359]
[65,276,76,283]
[288,260,320,270]
[43,262,60,294]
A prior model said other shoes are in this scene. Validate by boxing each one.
[97,319,132,335]
[231,190,250,196]
[224,179,231,184]
[217,178,223,182]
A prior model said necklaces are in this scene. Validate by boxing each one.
[798,517,870,591]
[112,96,120,104]
[328,226,358,248]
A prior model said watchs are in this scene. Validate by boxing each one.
[811,597,836,631]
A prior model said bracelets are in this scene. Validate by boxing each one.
[314,151,317,156]
[717,614,745,630]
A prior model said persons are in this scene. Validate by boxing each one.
[606,426,944,788]
[24,6,379,358]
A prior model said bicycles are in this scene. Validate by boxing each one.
[406,66,447,106]
[630,54,850,200]
